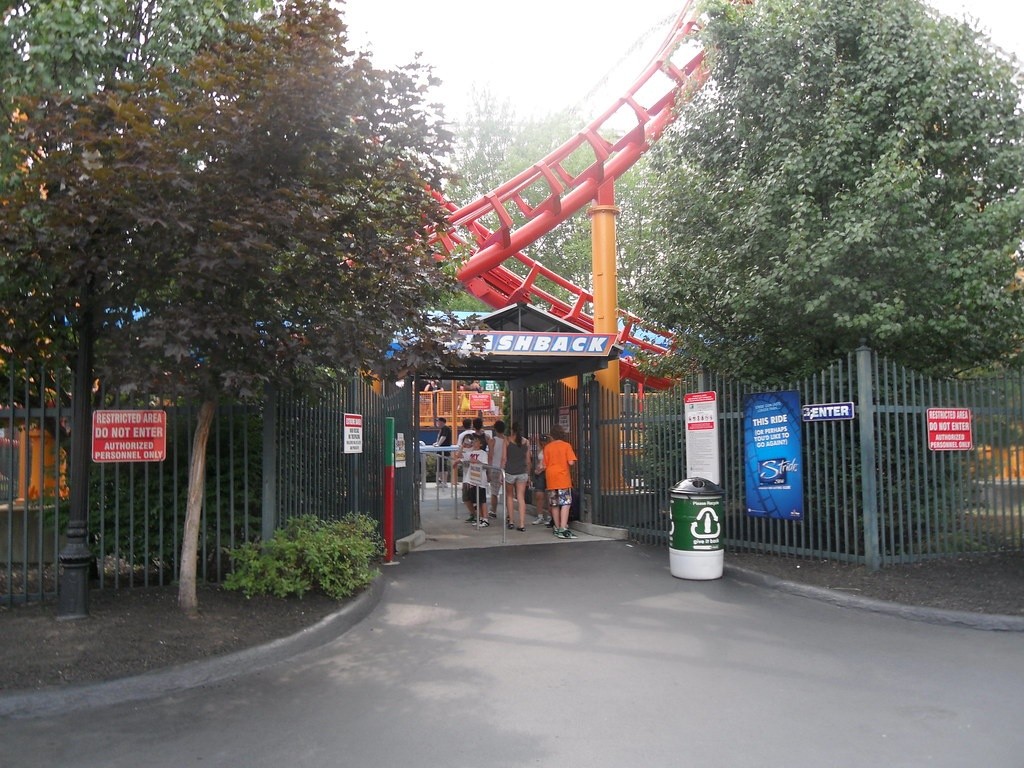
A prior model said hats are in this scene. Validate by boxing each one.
[472,433,486,450]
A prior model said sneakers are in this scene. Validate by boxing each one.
[478,518,489,528]
[532,513,545,525]
[543,515,553,528]
[557,527,578,539]
[487,510,497,519]
[471,516,482,526]
[471,516,478,522]
[506,516,510,523]
[552,525,561,536]
[464,514,474,523]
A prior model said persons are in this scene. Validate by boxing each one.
[421,377,488,412]
[541,424,579,539]
[457,436,491,528]
[499,423,532,532]
[456,418,476,447]
[471,418,491,450]
[433,417,452,489]
[452,434,479,524]
[486,420,513,526]
[532,433,556,528]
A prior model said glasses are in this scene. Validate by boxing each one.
[539,435,549,441]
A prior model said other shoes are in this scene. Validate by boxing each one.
[472,502,478,510]
[434,483,448,488]
[517,526,526,531]
[508,523,514,529]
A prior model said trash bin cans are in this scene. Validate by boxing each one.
[667,477,725,581]
